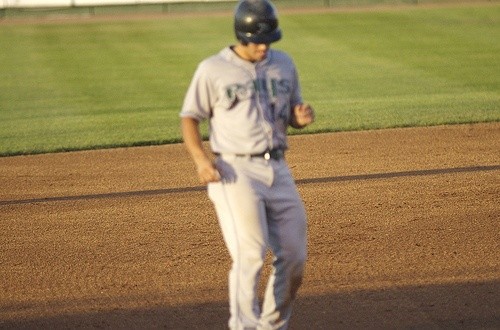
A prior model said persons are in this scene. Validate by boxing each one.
[177,0,316,330]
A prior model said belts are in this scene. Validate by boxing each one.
[212,148,285,161]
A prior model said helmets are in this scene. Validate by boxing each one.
[234,0,281,44]
